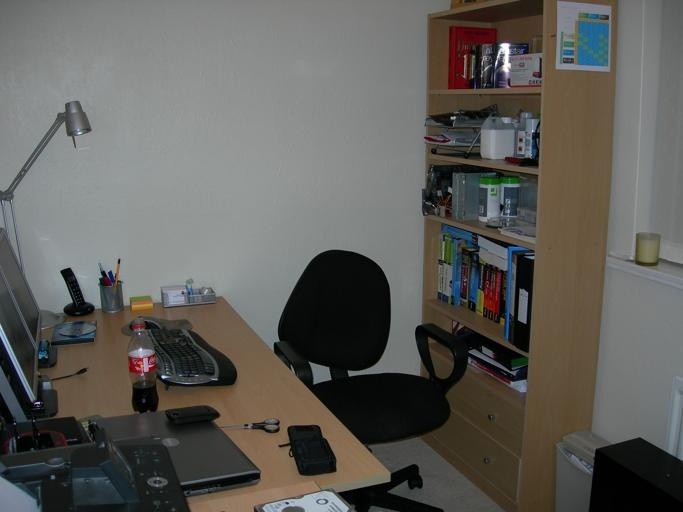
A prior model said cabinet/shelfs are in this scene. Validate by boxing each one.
[419,1,622,408]
[416,347,596,512]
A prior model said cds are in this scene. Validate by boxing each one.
[57,322,98,336]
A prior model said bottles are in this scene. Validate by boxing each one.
[128,318,159,414]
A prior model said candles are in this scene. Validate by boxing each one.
[637,233,660,264]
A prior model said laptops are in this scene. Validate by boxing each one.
[100,407,263,498]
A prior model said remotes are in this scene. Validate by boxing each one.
[119,442,193,512]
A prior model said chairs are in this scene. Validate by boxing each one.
[270,247,471,512]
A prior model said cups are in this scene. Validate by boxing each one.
[635,231,661,267]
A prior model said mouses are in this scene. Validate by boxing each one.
[130,319,162,330]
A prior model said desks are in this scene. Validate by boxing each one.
[36,296,392,512]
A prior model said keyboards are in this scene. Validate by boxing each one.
[146,326,238,389]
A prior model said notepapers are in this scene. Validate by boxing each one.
[130,295,154,311]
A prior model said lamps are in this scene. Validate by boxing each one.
[2,101,90,203]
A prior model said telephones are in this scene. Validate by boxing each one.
[60,267,95,316]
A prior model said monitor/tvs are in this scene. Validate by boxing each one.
[0,222,56,367]
[0,251,58,419]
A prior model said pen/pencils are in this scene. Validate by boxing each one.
[97,258,121,308]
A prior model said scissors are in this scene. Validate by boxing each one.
[217,418,280,432]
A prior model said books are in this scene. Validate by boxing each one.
[437,223,535,353]
[448,26,543,89]
[498,226,536,245]
[468,342,528,385]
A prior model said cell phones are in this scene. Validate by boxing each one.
[165,405,220,424]
